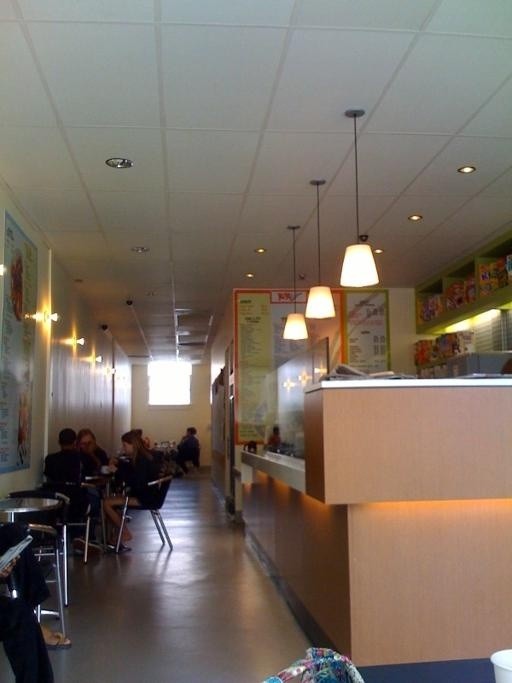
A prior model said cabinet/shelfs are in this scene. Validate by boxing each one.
[415,229,512,379]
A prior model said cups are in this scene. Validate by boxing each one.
[101,465,111,474]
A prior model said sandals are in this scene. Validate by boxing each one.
[40,625,72,650]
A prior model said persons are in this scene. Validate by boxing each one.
[101,429,159,546]
[130,427,201,478]
[76,427,113,478]
[0,521,72,682]
[43,427,133,556]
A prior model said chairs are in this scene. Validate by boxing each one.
[140,442,200,479]
[0,441,121,651]
[115,476,172,554]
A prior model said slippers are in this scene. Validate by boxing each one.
[106,543,132,555]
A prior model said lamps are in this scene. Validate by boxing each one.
[339,108,381,289]
[304,177,337,320]
[282,225,309,340]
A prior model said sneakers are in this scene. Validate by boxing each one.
[72,536,103,555]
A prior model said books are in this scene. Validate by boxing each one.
[414,252,512,377]
[316,362,418,381]
